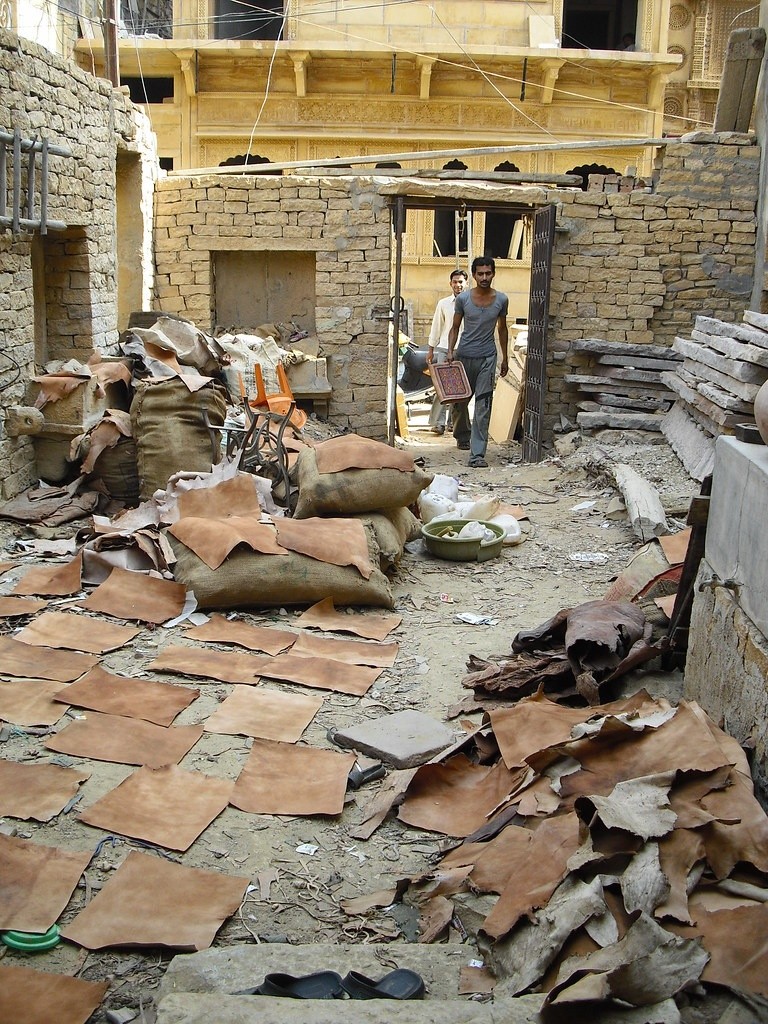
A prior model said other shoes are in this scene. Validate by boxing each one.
[469,459,488,467]
[431,426,445,434]
[448,427,453,431]
[457,442,470,450]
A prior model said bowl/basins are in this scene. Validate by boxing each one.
[421,519,508,562]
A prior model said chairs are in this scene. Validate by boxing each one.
[236,362,308,431]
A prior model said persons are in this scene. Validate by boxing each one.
[447,257,508,469]
[425,269,469,435]
